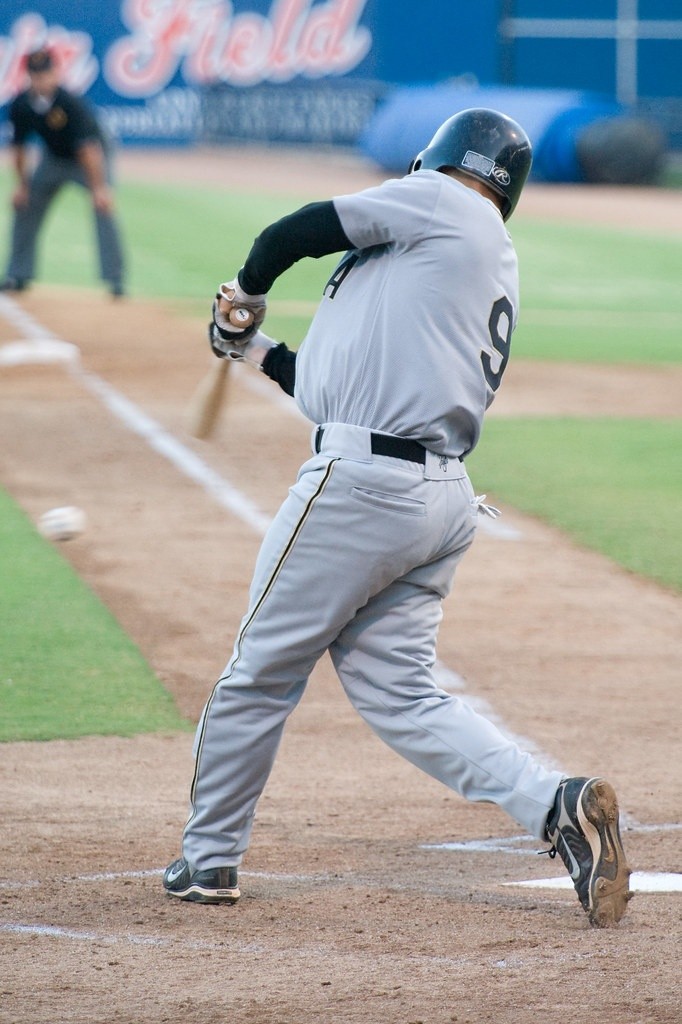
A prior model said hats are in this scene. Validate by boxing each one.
[25,50,55,71]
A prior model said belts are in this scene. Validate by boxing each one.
[316,429,463,466]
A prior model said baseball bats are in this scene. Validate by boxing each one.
[192,306,255,442]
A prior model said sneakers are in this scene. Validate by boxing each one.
[538,776,634,928]
[162,856,241,906]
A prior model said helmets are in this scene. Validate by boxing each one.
[408,107,533,222]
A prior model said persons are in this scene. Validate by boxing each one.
[0,50,125,296]
[163,109,634,928]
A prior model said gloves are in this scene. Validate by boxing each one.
[211,275,267,344]
[208,321,278,375]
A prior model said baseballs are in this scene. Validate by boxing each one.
[36,505,86,542]
